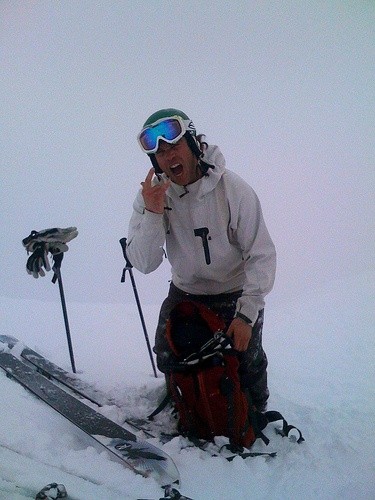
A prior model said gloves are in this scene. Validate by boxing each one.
[22,224,78,279]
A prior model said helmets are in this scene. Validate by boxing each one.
[141,109,201,171]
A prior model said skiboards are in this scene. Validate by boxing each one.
[0,330,279,500]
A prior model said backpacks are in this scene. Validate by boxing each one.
[168,300,265,451]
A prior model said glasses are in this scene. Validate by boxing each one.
[137,114,194,153]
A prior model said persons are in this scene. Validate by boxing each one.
[125,108,277,454]
